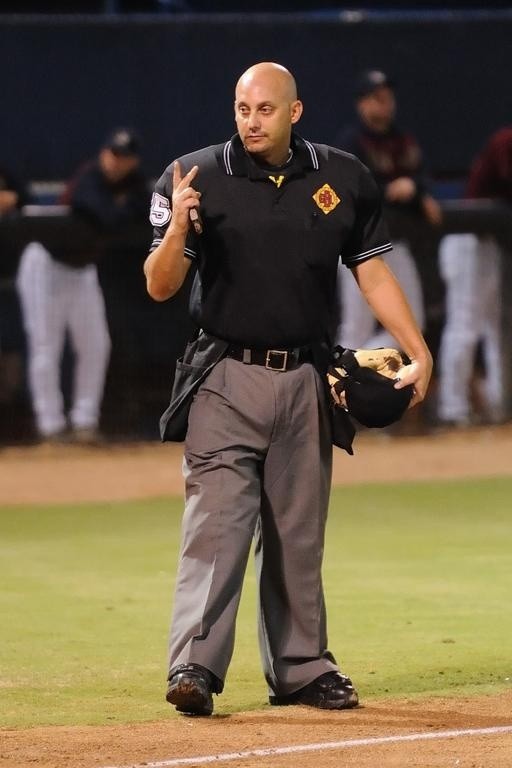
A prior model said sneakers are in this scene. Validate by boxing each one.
[269,671,358,709]
[166,664,213,716]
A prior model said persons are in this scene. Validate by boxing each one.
[334,67,441,356]
[432,126,512,429]
[145,61,435,715]
[14,127,144,447]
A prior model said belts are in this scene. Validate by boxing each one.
[226,343,309,372]
